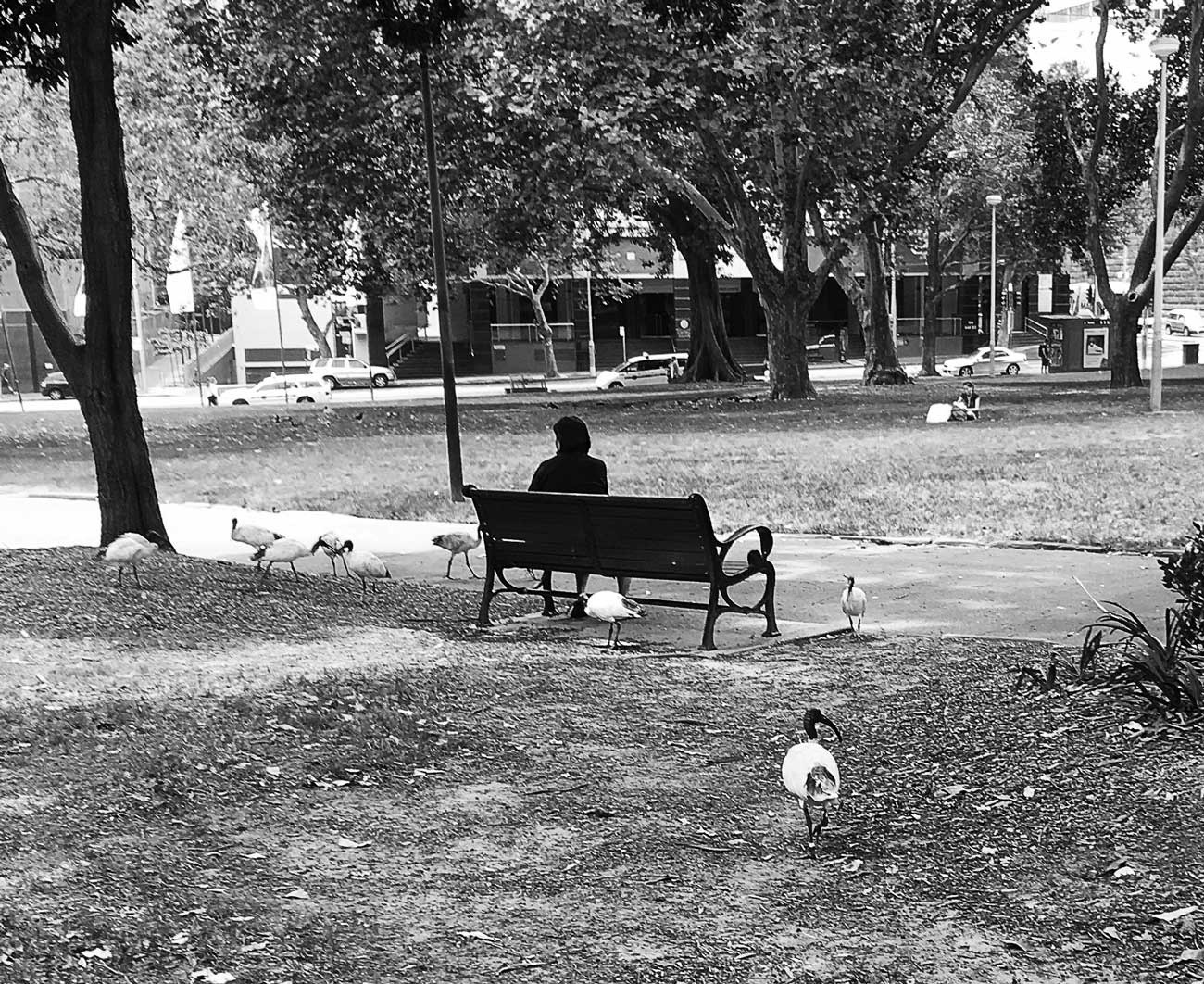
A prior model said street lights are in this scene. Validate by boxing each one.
[1111,37,1150,372]
[984,195,1002,378]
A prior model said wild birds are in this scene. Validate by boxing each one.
[97,532,159,590]
[568,590,647,648]
[781,706,842,852]
[433,525,486,580]
[231,518,391,595]
[841,576,868,632]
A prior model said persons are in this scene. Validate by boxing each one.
[1040,339,1050,375]
[763,355,769,370]
[1,363,9,394]
[952,382,981,420]
[667,357,680,377]
[529,415,647,618]
[839,329,846,363]
[206,377,217,406]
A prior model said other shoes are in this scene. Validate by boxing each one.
[636,608,647,616]
[571,602,585,617]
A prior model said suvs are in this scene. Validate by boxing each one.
[307,357,395,390]
[279,405,279,406]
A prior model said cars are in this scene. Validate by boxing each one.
[943,345,1028,376]
[597,352,689,391]
[225,374,332,406]
[1165,309,1204,337]
[39,371,77,400]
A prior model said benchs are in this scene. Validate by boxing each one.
[463,483,780,650]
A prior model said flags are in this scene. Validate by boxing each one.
[239,198,274,285]
[166,207,196,314]
[72,261,86,317]
